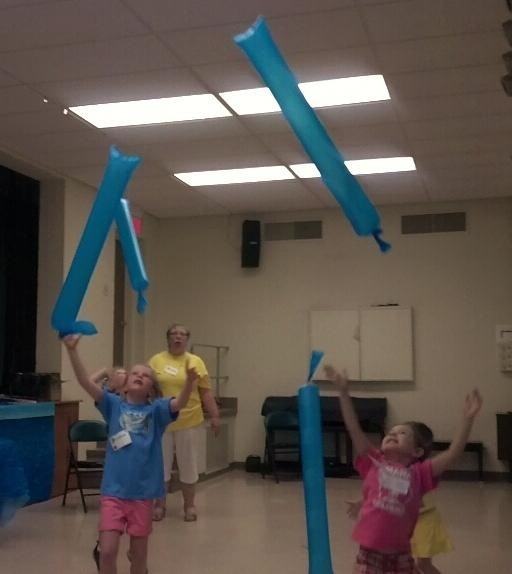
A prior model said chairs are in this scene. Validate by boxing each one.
[62,418,108,513]
[262,410,302,484]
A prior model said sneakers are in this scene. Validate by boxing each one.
[149,506,198,522]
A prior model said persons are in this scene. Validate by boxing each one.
[58,333,201,572]
[323,360,483,573]
[100,366,129,393]
[345,489,456,573]
[149,324,223,523]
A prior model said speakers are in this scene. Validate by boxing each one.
[241,219,260,267]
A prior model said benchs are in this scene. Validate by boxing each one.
[419,440,484,481]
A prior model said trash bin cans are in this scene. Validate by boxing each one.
[496,410,512,463]
